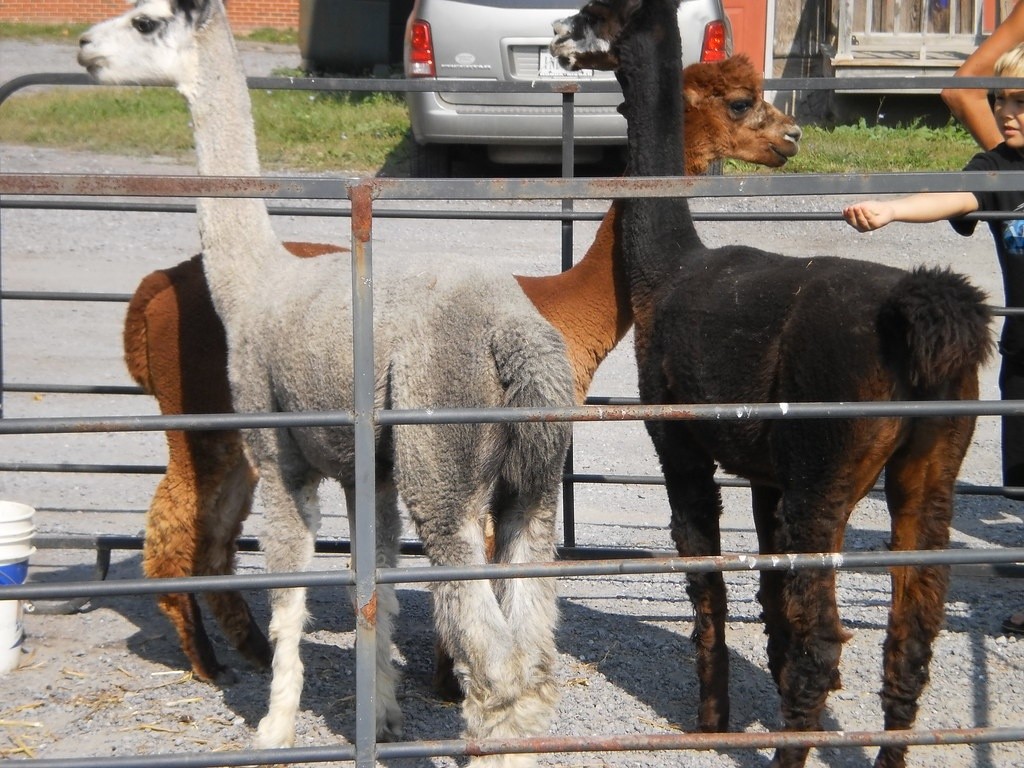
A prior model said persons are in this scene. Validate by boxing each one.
[939,0,1024,153]
[842,38,1024,503]
